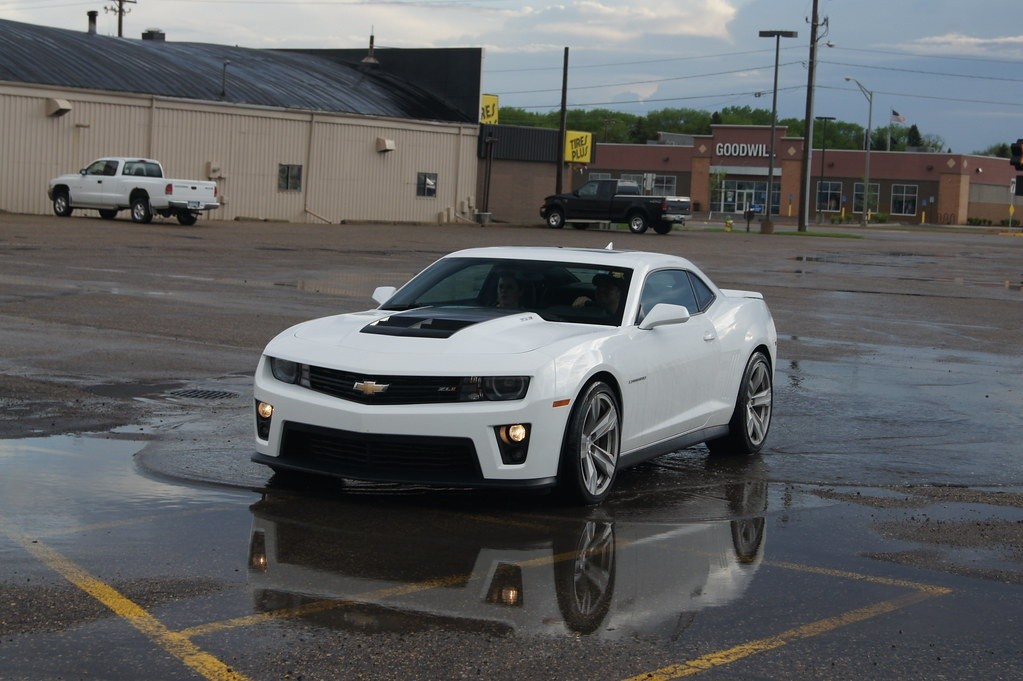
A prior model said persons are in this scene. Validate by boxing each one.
[572,270,644,326]
[493,272,523,310]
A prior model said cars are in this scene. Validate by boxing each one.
[244,472,766,640]
[251,240,781,508]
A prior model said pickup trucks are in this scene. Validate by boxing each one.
[47,158,220,227]
[540,178,693,235]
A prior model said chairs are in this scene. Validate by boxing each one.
[134,168,143,176]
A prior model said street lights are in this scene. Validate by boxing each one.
[760,30,799,220]
[805,42,834,225]
[816,116,836,214]
[846,77,873,226]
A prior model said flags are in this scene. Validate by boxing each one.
[892,110,906,123]
